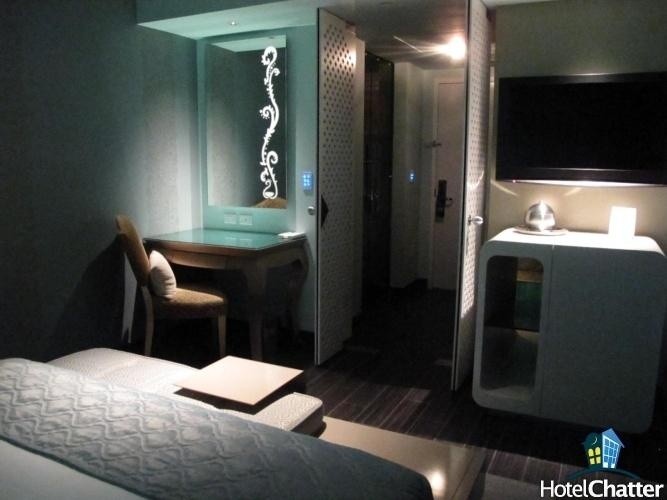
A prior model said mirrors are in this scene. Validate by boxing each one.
[204,33,289,209]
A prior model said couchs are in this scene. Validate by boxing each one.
[0,347,488,500]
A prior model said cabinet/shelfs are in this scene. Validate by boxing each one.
[470,226,667,435]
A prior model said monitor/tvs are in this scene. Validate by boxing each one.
[497,71,667,186]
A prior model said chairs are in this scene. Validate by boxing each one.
[114,214,229,361]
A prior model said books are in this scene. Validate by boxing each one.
[175,355,306,411]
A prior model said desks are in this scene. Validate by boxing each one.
[142,229,308,364]
